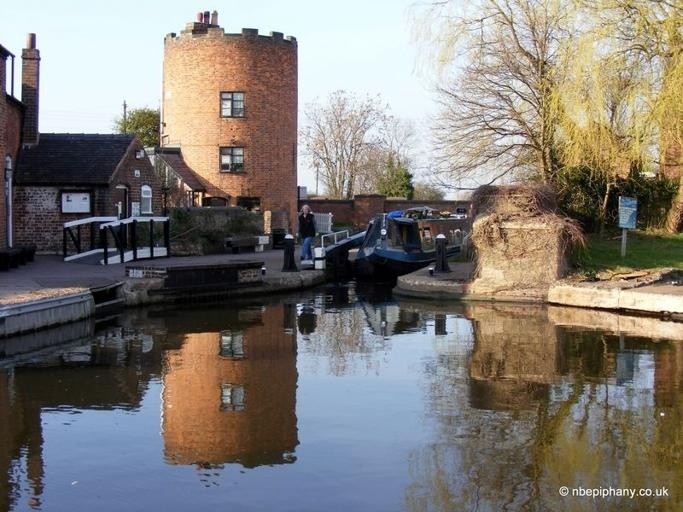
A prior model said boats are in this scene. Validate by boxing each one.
[355,211,461,289]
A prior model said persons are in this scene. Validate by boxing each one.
[298,205,316,261]
